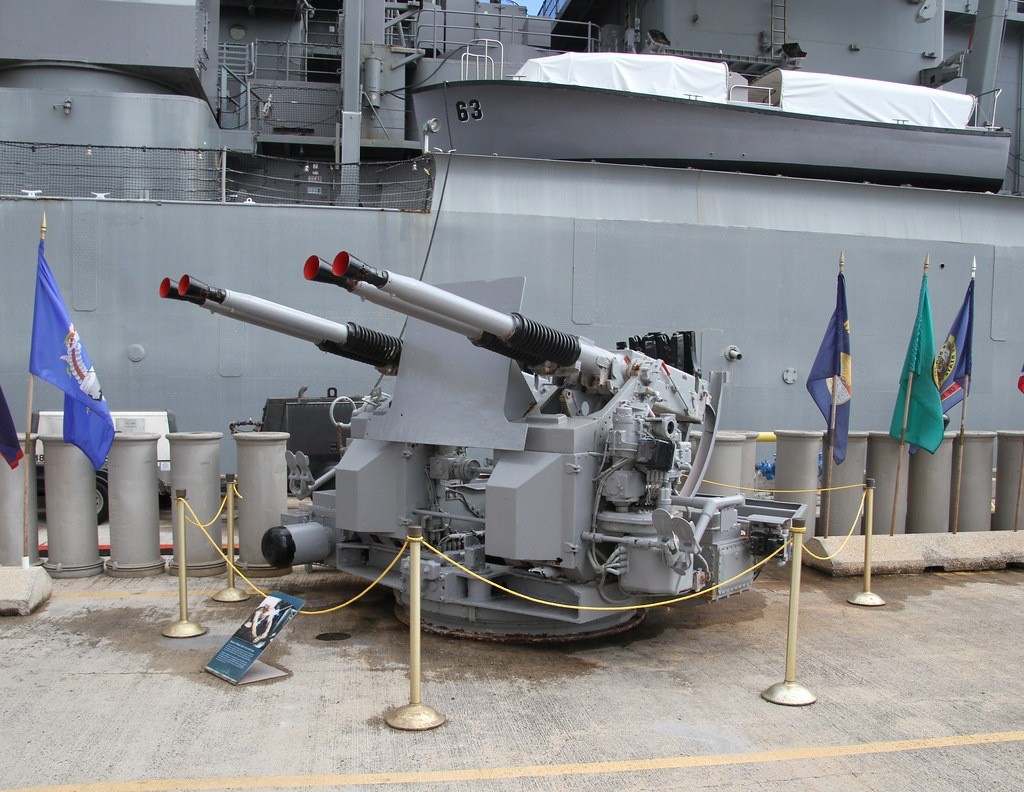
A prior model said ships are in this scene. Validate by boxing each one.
[2,0,1023,493]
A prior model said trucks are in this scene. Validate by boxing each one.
[36,390,373,529]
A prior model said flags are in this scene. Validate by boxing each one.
[908,278,976,455]
[0,384,24,470]
[27,237,115,474]
[888,274,944,455]
[805,270,854,466]
